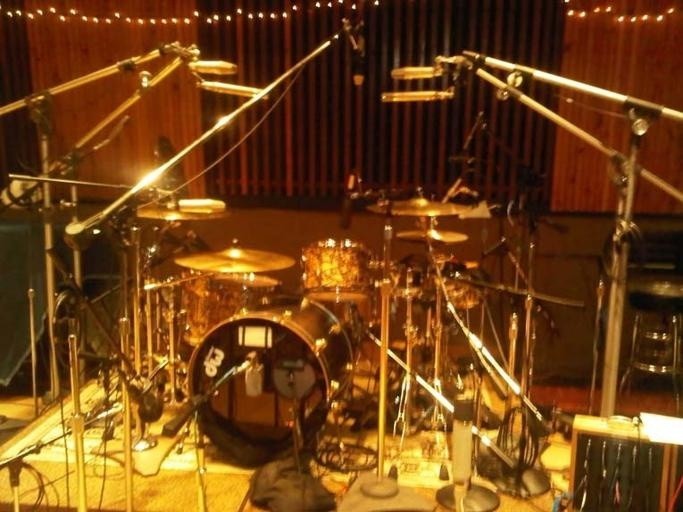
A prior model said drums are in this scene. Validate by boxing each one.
[300,238,369,302]
[183,273,282,347]
[187,295,357,464]
[143,232,210,304]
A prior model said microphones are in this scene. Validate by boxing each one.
[480,235,510,258]
[188,60,237,75]
[199,78,271,101]
[378,87,455,105]
[338,14,366,52]
[168,198,227,214]
[389,65,446,82]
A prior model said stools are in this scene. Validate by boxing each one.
[619,277,683,401]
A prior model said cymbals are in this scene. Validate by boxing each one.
[396,231,467,244]
[368,198,471,216]
[136,199,230,220]
[173,248,295,273]
